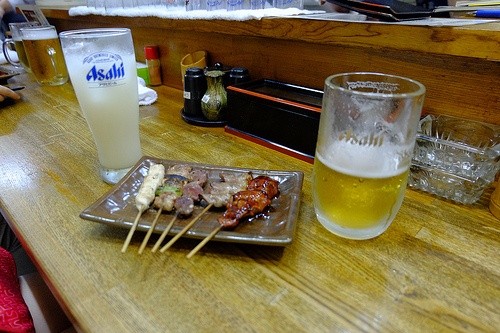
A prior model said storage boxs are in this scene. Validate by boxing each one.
[224,77,324,165]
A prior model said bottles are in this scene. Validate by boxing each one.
[145,45,163,86]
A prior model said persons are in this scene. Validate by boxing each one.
[0,85,20,102]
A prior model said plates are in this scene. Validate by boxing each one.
[327,0,434,22]
[79,155,304,246]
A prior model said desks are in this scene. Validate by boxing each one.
[1,1,500,333]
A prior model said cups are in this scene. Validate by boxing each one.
[20,26,69,87]
[313,72,425,239]
[3,22,40,73]
[59,28,143,185]
